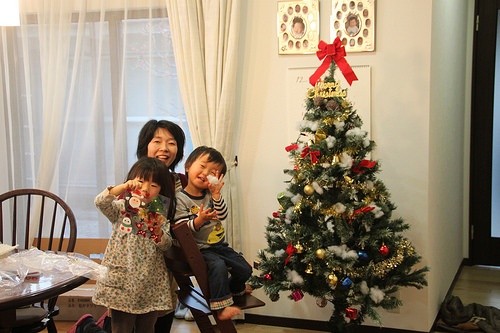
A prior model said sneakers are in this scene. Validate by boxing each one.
[175,302,194,321]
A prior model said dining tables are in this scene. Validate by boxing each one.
[0,249,92,333]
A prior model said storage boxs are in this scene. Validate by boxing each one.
[43,280,108,322]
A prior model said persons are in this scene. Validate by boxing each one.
[281,4,317,50]
[334,0,371,47]
[68,157,181,333]
[117,119,189,333]
[170,146,253,321]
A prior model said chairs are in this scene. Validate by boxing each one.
[0,189,77,333]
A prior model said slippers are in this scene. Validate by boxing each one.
[95,310,110,329]
[67,314,93,333]
